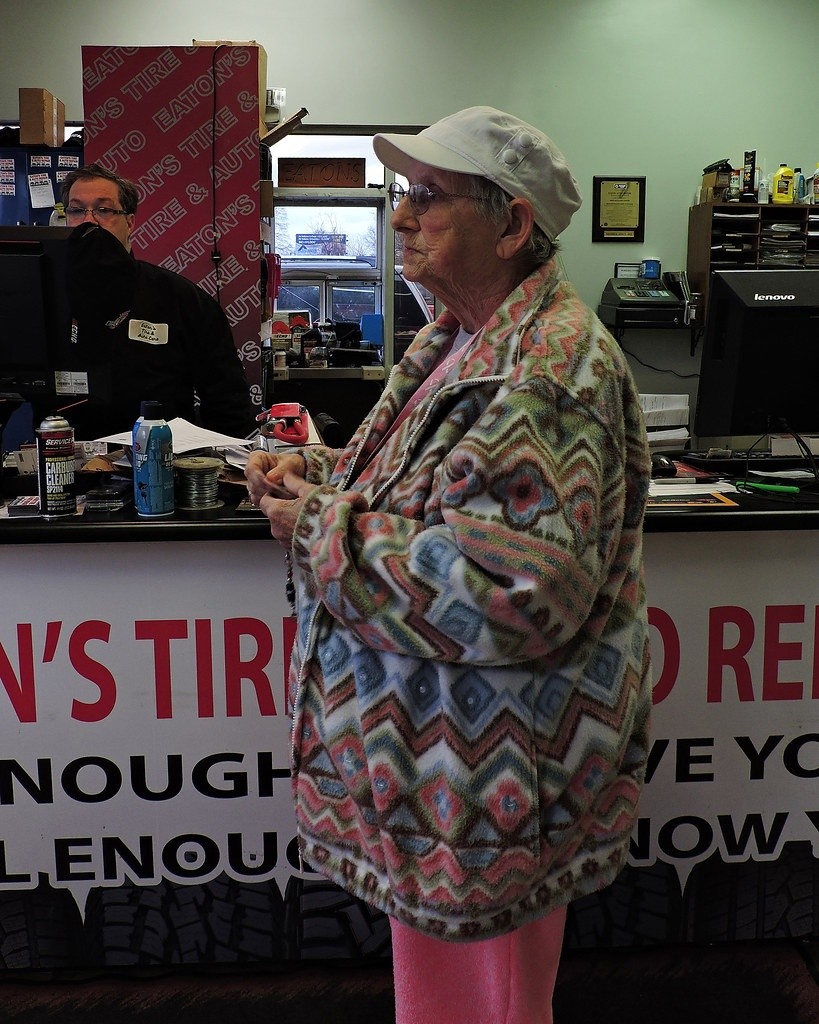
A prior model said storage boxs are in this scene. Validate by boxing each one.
[700,172,730,203]
[18,88,66,149]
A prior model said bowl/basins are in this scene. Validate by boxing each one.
[311,317,337,345]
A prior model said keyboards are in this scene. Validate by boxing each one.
[680,452,819,472]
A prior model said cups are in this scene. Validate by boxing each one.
[271,333,291,350]
[293,333,306,355]
[309,346,329,367]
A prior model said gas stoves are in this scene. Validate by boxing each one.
[330,337,379,367]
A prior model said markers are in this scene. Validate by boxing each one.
[655,477,719,485]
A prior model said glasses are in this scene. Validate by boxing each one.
[388,182,512,215]
[62,206,129,221]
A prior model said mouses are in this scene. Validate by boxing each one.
[650,455,677,476]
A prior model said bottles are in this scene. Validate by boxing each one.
[772,163,793,204]
[793,168,808,202]
[758,177,770,204]
[300,322,323,367]
[729,169,740,200]
[34,415,77,517]
[132,402,176,516]
[813,163,819,205]
[273,348,287,370]
[641,256,660,279]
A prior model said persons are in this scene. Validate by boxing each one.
[62,167,257,439]
[245,106,652,1024]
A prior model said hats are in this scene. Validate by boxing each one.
[373,106,583,244]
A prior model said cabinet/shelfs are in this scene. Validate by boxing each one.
[81,38,268,417]
[686,203,818,331]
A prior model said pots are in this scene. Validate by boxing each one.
[326,315,362,340]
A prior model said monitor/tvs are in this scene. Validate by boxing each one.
[693,269,819,436]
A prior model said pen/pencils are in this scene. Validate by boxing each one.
[736,481,799,493]
